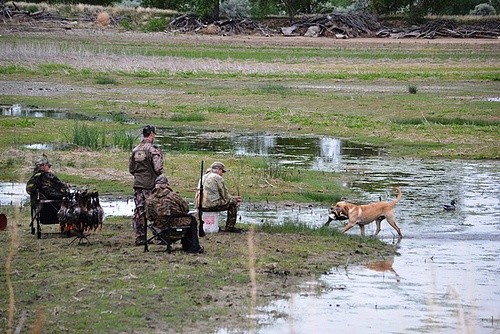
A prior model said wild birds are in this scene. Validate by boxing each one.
[58,186,105,232]
[324,207,349,227]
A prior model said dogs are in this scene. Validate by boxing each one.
[329,186,402,244]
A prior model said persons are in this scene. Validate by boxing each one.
[194,161,244,232]
[27,155,69,200]
[129,124,204,253]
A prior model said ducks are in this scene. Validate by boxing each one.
[442,200,457,211]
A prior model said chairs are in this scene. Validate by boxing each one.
[140,195,192,253]
[28,187,63,239]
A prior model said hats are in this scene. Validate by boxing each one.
[211,162,226,173]
[35,158,53,166]
[155,175,169,184]
[143,125,157,135]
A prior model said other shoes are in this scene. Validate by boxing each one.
[225,227,241,233]
[198,247,204,253]
